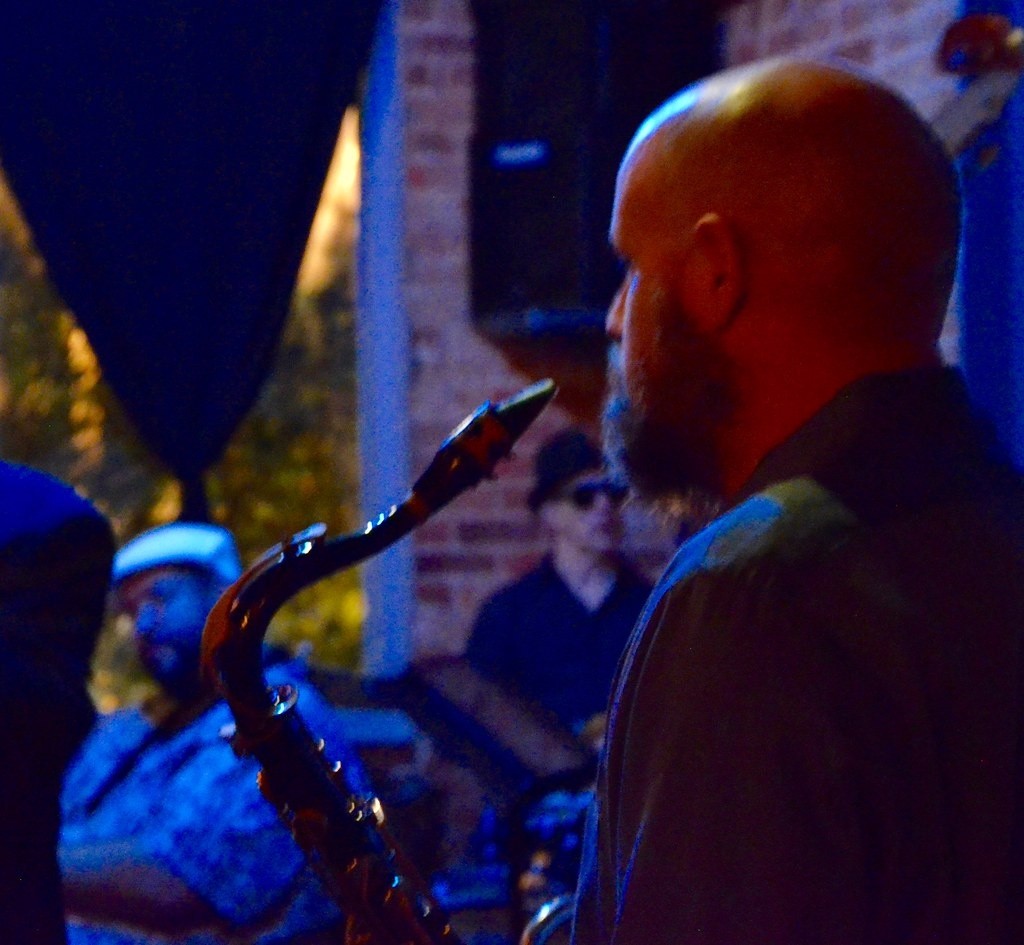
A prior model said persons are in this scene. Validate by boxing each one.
[0,424,658,945]
[523,48,1024,945]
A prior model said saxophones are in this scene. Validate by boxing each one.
[196,375,557,943]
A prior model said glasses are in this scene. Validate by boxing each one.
[557,480,627,507]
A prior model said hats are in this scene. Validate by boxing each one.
[110,521,242,585]
[526,434,615,511]
